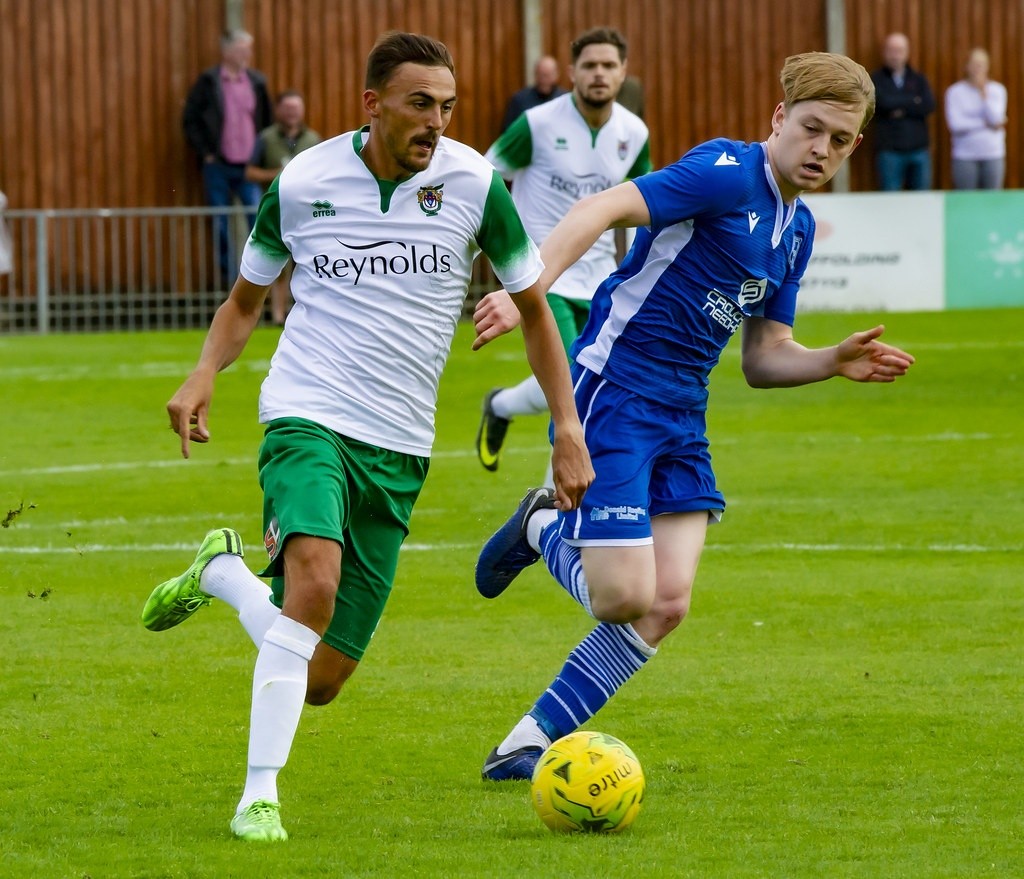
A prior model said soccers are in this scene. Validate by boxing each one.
[529,730,646,833]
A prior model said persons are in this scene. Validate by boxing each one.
[870,33,937,192]
[245,91,324,320]
[945,48,1008,191]
[475,27,655,473]
[181,27,274,291]
[504,56,567,133]
[142,33,597,842]
[472,51,915,780]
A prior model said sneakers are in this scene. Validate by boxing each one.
[475,485,561,598]
[231,796,287,844]
[140,526,244,632]
[475,388,512,473]
[480,745,545,781]
